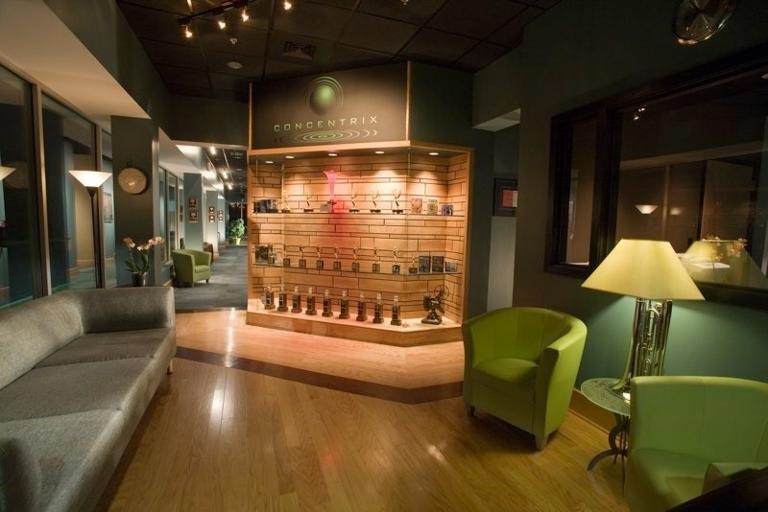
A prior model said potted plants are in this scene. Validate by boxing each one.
[227,219,245,245]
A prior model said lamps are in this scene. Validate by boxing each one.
[684,240,768,291]
[68,169,113,287]
[118,161,147,194]
[580,238,705,402]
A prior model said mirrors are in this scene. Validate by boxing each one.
[543,43,768,311]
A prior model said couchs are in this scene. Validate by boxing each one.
[0,286,178,512]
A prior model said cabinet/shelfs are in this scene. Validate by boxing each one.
[246,211,467,347]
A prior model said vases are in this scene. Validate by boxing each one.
[133,272,149,286]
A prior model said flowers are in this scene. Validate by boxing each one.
[123,236,162,273]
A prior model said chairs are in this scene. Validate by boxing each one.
[461,307,588,450]
[171,249,212,287]
[624,375,768,510]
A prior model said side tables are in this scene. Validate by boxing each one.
[581,377,630,496]
